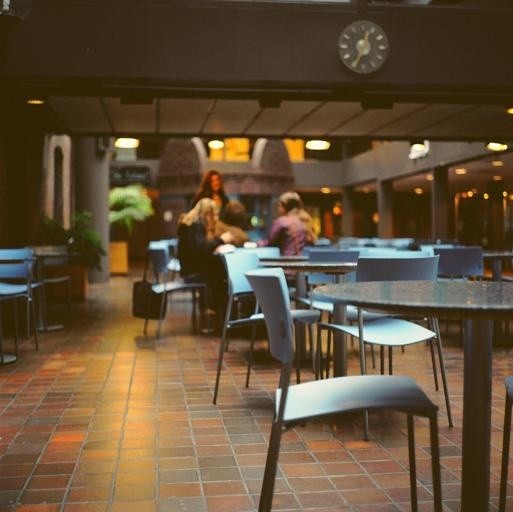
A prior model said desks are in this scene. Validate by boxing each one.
[312,273,510,511]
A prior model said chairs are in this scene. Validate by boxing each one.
[302,249,365,313]
[208,231,440,260]
[211,248,319,410]
[26,245,77,334]
[244,268,322,392]
[147,233,205,337]
[0,245,38,363]
[431,245,486,280]
[235,267,446,512]
[324,253,462,443]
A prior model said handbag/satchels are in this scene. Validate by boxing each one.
[133,282,167,319]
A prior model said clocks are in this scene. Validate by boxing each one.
[337,20,391,76]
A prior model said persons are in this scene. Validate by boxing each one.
[258,191,318,285]
[173,168,251,338]
